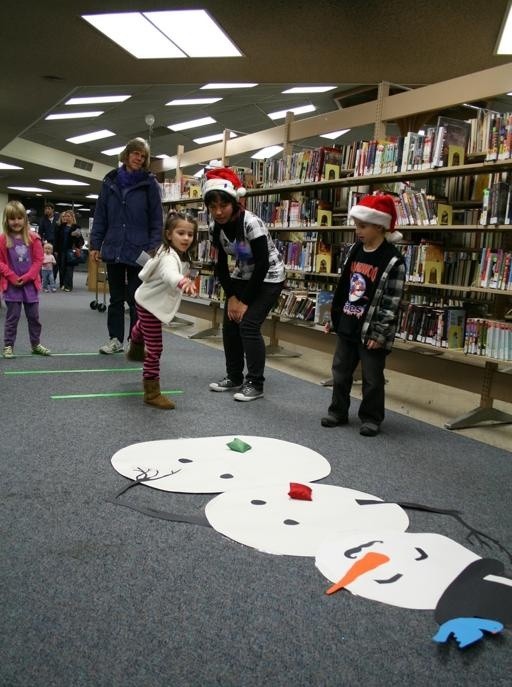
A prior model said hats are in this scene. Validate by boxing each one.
[349,195,404,242]
[199,167,247,203]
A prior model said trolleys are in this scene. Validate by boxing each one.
[91,261,109,312]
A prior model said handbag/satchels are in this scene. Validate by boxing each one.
[67,240,88,264]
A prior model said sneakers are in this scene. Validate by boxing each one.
[99,337,124,354]
[4,345,15,358]
[43,288,49,292]
[209,377,242,391]
[321,413,349,427]
[233,383,264,401]
[32,343,51,355]
[359,420,379,436]
[51,287,57,292]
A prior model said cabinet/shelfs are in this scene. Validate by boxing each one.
[159,109,511,375]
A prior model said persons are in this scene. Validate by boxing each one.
[321,196,407,437]
[201,167,286,402]
[124,211,198,409]
[0,200,51,358]
[36,203,84,292]
[89,137,163,355]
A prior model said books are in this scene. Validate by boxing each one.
[152,109,510,363]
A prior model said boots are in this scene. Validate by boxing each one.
[127,341,146,361]
[144,379,176,409]
